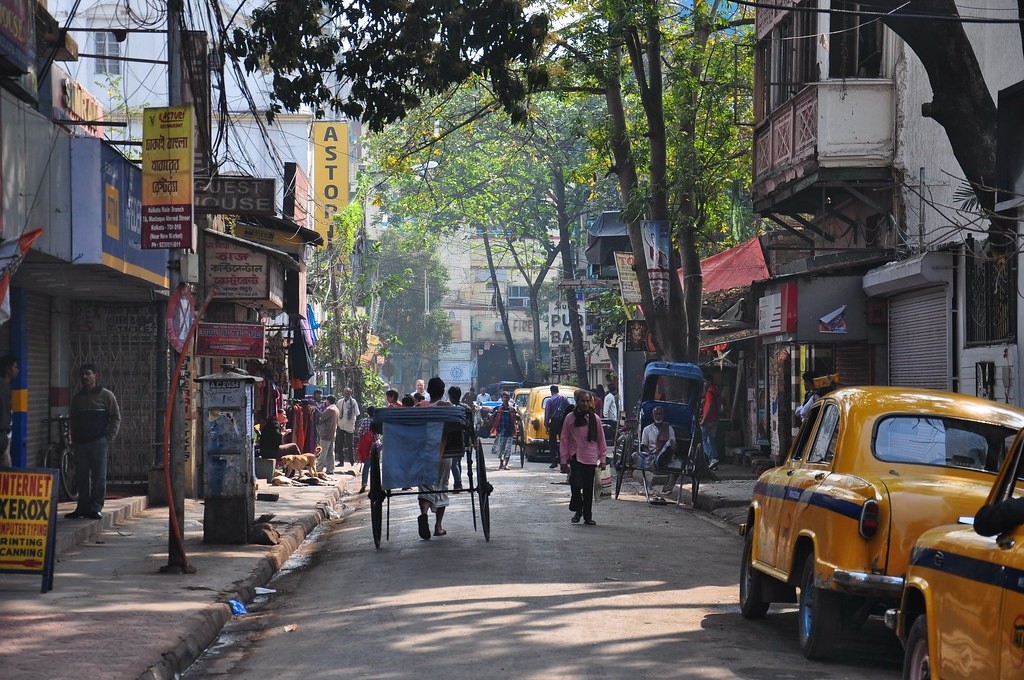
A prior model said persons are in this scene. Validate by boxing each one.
[401,394,415,408]
[413,393,425,404]
[335,387,361,467]
[476,387,492,402]
[461,387,477,408]
[560,389,607,524]
[700,374,722,467]
[259,408,303,477]
[358,405,382,494]
[800,371,832,429]
[448,386,472,410]
[543,384,570,468]
[386,390,403,407]
[413,377,446,406]
[590,374,618,420]
[63,365,121,520]
[310,388,339,475]
[631,405,678,470]
[488,391,522,469]
[410,378,430,400]
[0,355,20,467]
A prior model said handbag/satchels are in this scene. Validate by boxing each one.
[595,463,613,503]
[557,399,567,422]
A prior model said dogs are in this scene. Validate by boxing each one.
[281,446,322,477]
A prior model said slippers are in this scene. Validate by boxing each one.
[418,514,431,539]
[434,527,446,536]
[572,512,582,523]
[584,520,596,526]
[651,497,666,506]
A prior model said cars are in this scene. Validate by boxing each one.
[505,385,597,462]
[740,385,1024,680]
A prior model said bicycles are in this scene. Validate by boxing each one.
[41,415,82,500]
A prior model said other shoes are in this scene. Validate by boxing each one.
[64,506,87,518]
[549,462,557,468]
[709,458,720,471]
[499,465,503,468]
[88,510,101,519]
[505,466,511,470]
[360,485,367,493]
[335,463,344,467]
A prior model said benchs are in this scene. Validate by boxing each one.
[642,424,689,443]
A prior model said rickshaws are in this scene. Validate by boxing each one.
[367,403,493,551]
[473,399,520,440]
[613,359,712,509]
